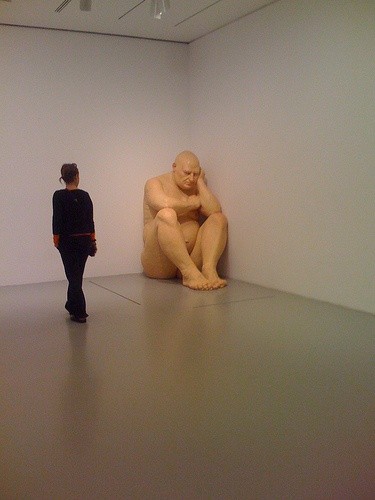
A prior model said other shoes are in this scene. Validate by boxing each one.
[70,313,88,323]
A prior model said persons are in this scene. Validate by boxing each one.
[52,162,97,323]
[141,151,229,290]
